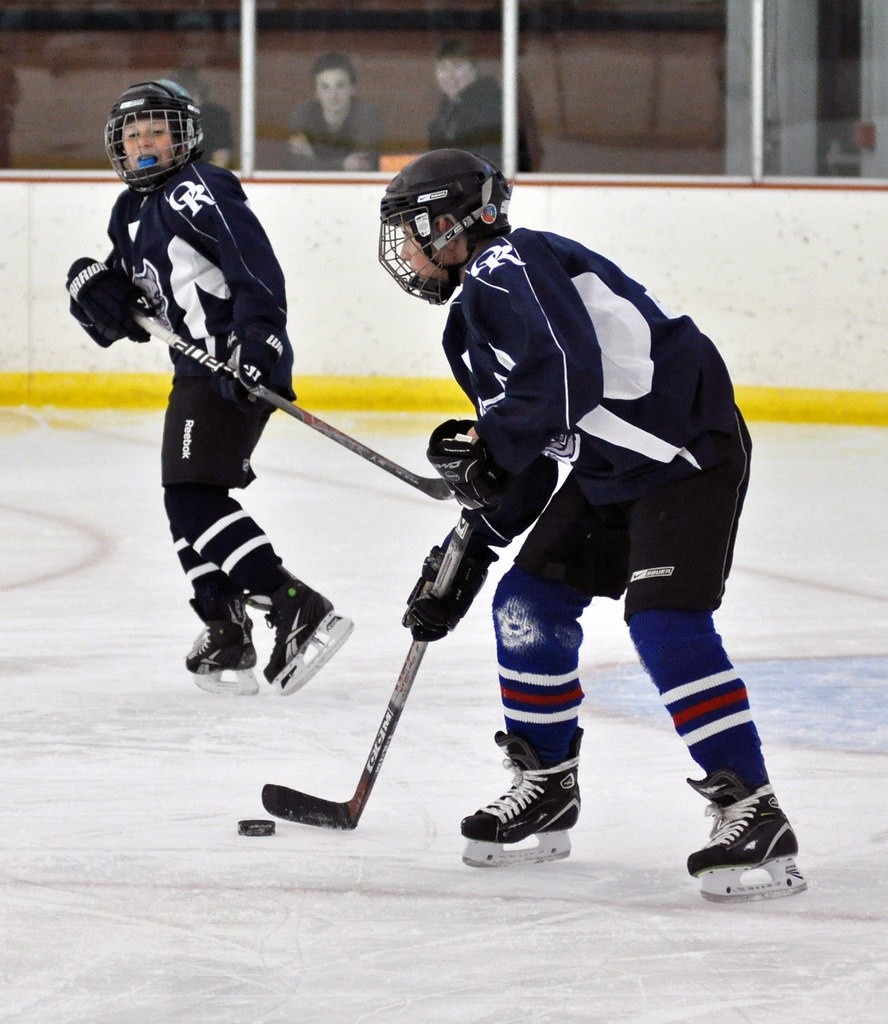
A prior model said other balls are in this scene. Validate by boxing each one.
[237,818,275,837]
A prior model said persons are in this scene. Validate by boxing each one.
[65,79,334,689]
[164,67,234,170]
[427,37,533,173]
[377,147,799,879]
[285,53,383,172]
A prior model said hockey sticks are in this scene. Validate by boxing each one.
[262,508,475,831]
[139,316,454,502]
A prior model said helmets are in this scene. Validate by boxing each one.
[379,148,511,307]
[103,79,206,193]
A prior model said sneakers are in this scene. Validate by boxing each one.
[186,600,260,696]
[240,564,354,696]
[461,726,585,869]
[686,773,809,903]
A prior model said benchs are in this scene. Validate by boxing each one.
[2,25,724,174]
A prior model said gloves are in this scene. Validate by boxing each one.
[225,322,285,390]
[64,256,155,343]
[401,526,499,643]
[427,418,509,517]
